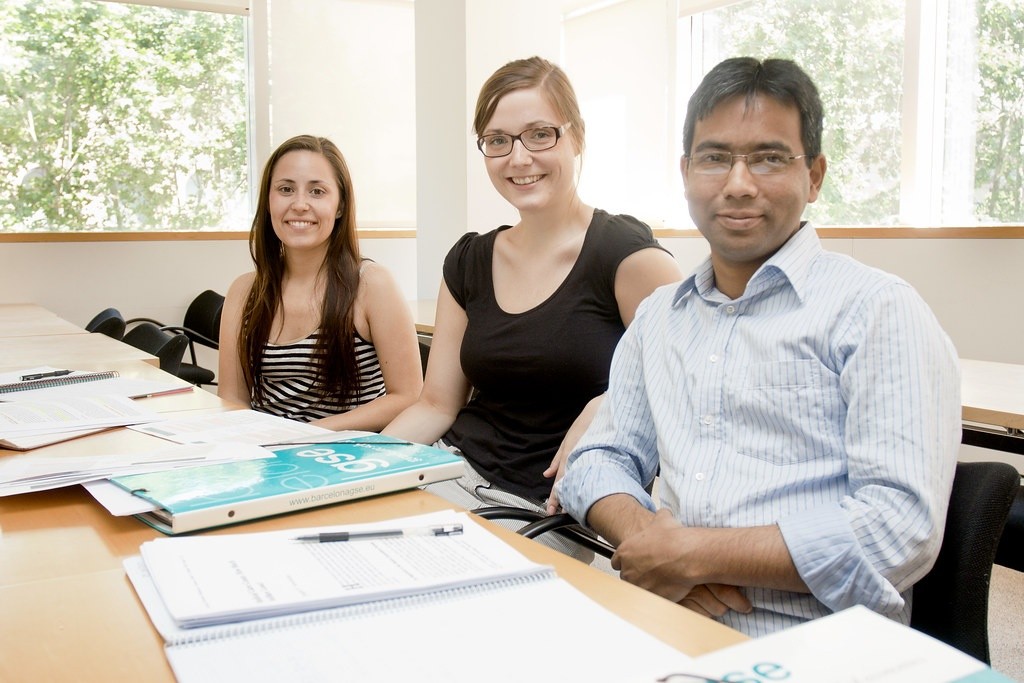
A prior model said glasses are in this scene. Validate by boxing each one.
[476,122,572,158]
[687,151,818,175]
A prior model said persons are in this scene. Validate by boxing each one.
[556,60,962,639]
[219,137,425,431]
[381,56,685,563]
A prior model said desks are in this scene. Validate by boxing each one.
[1,333,158,369]
[960,356,1024,455]
[2,305,83,335]
[2,362,752,683]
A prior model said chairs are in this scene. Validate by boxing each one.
[86,308,124,341]
[519,463,1017,667]
[126,290,225,388]
[123,323,188,377]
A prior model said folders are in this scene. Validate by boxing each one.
[103,429,465,536]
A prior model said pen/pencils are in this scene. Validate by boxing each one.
[134,387,193,400]
[293,529,405,545]
[18,368,75,381]
